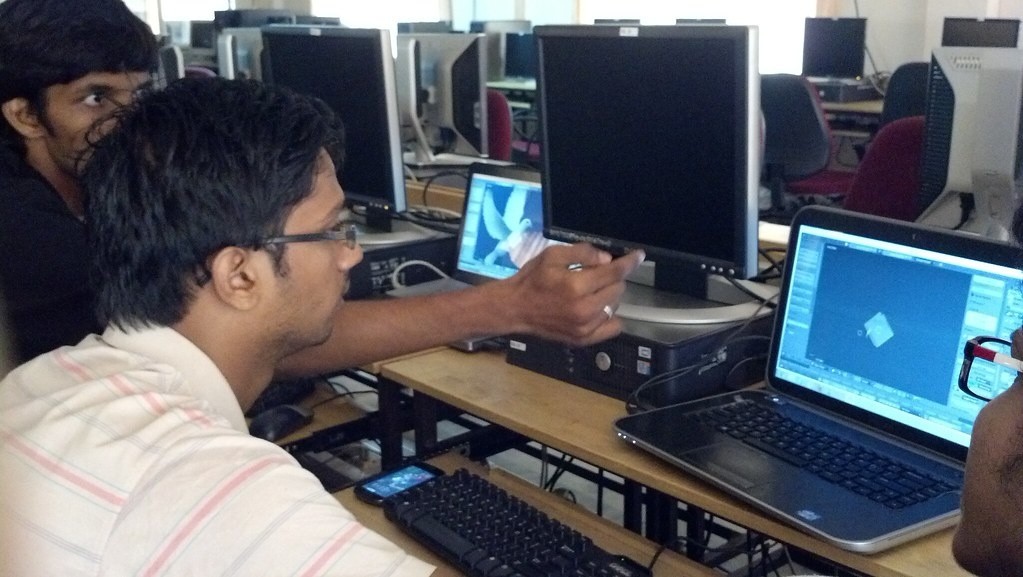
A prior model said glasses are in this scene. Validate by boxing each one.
[235,208,356,251]
[957,336,1023,402]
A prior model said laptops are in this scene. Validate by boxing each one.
[386,162,585,353]
[611,206,1023,556]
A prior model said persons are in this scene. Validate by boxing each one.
[950,324,1023,577]
[0,0,645,376]
[0,76,437,577]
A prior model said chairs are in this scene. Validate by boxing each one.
[843,111,928,219]
[753,73,852,216]
[486,87,540,167]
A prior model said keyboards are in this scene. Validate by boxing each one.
[244,378,316,418]
[383,466,654,577]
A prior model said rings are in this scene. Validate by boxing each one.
[604,305,612,320]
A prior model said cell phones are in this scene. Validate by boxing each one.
[354,459,446,506]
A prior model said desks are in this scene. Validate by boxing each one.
[244,179,978,577]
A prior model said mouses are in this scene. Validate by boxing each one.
[249,404,315,443]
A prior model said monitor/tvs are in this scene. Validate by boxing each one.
[154,10,1023,325]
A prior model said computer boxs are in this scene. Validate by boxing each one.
[343,231,458,303]
[815,84,880,102]
[505,284,782,413]
